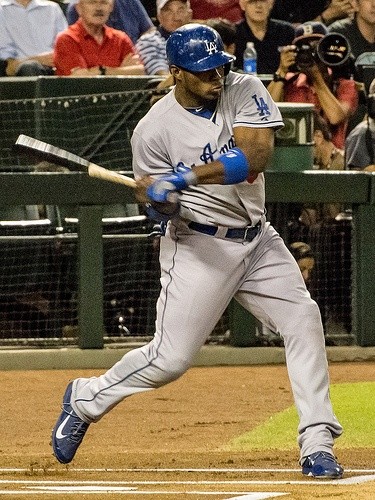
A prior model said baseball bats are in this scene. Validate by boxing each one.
[15,134,180,203]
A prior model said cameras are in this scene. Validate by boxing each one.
[288,44,320,69]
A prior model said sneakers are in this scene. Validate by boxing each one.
[302,453,342,479]
[50,384,90,464]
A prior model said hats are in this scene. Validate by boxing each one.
[291,22,329,44]
[156,0,191,13]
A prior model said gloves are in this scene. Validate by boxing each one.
[148,170,190,206]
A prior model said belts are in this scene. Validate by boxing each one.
[187,220,262,242]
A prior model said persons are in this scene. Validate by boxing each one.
[0,0,375,227]
[52,23,346,480]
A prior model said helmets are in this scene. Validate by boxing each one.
[165,24,236,72]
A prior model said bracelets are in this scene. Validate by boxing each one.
[146,205,175,222]
[99,65,106,75]
[218,147,249,186]
[273,71,286,85]
